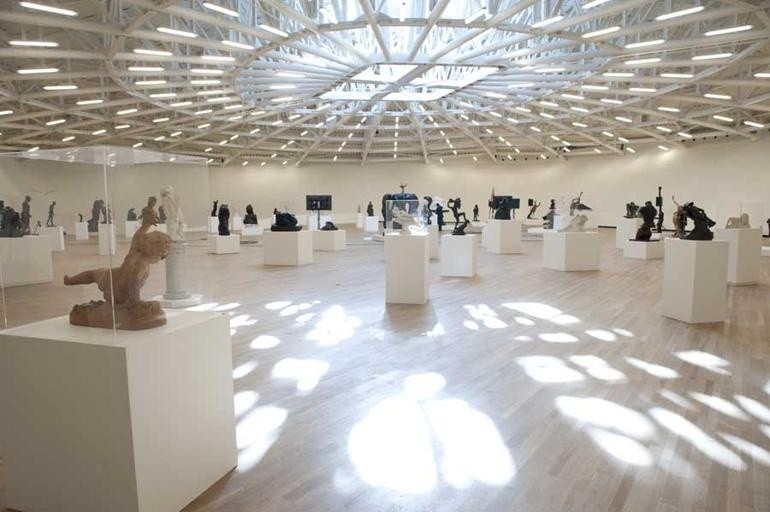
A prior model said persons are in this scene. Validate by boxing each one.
[0,184,185,238]
[63,213,171,302]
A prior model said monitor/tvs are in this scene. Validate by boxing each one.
[307,195,332,210]
